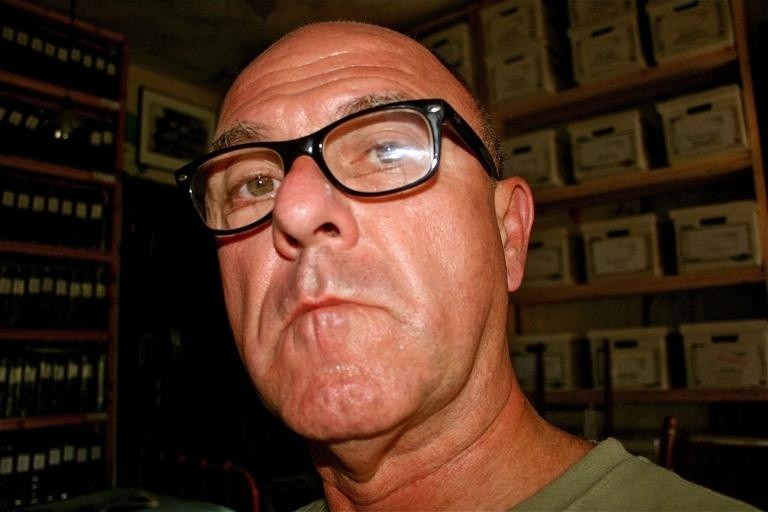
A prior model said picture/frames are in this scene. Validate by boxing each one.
[135,82,219,176]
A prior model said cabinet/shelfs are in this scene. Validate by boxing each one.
[404,0,767,405]
[1,1,131,511]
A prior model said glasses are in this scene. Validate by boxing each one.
[172,98,499,238]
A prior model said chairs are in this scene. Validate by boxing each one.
[661,416,768,512]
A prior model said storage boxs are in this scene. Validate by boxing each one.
[570,2,647,88]
[508,331,576,391]
[518,227,572,284]
[648,1,736,65]
[670,201,763,276]
[479,1,556,105]
[656,84,749,165]
[568,111,647,181]
[579,214,661,282]
[679,319,766,390]
[420,23,473,91]
[498,129,562,191]
[586,325,668,390]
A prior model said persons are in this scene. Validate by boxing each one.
[175,21,767,511]
